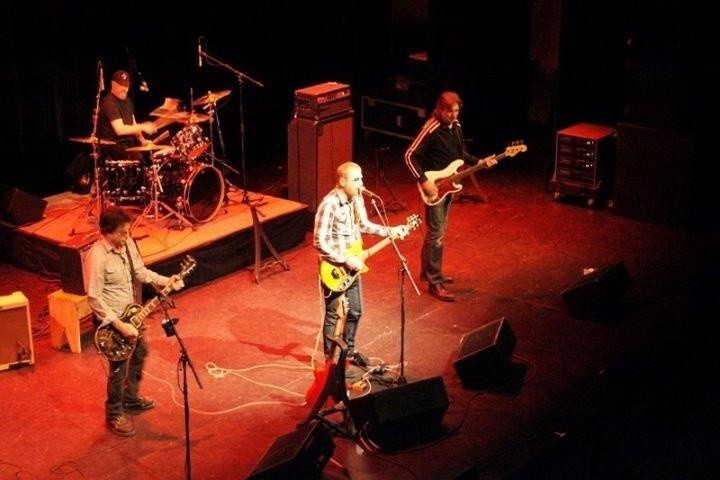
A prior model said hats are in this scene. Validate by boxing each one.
[111,70,132,87]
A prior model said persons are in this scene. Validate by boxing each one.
[95,70,158,161]
[403,89,498,301]
[85,206,185,438]
[313,162,410,378]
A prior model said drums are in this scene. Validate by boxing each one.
[169,124,211,161]
[106,159,144,199]
[168,161,225,223]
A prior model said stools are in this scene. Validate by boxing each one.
[46,289,93,353]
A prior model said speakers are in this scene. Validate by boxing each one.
[0,184,47,227]
[558,261,629,320]
[245,419,337,480]
[344,374,451,450]
[58,228,103,296]
[451,315,518,388]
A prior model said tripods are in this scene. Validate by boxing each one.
[202,53,265,217]
[128,194,198,239]
[367,197,421,386]
[69,184,114,237]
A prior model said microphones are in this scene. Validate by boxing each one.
[143,274,160,296]
[358,184,380,198]
[98,66,106,92]
[198,43,203,67]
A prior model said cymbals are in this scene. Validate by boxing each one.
[176,114,209,123]
[127,144,170,151]
[193,90,230,106]
[70,134,116,146]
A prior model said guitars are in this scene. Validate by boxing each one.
[419,141,528,206]
[319,214,422,293]
[94,254,197,362]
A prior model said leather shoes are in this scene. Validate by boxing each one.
[108,416,135,437]
[428,282,456,301]
[121,396,156,410]
[419,272,455,288]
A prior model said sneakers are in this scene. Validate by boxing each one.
[346,351,368,368]
